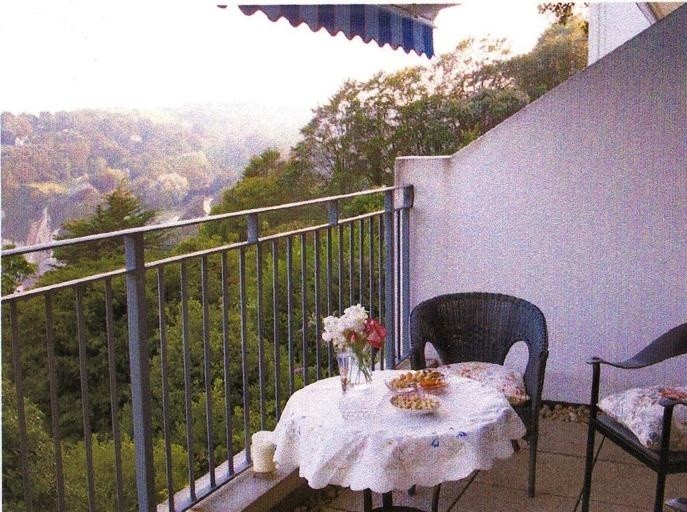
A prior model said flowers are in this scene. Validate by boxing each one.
[320,303,385,383]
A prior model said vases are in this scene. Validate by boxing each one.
[335,351,377,394]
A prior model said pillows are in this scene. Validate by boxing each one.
[595,384,687,452]
[428,362,530,405]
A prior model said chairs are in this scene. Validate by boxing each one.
[581,323,687,511]
[409,290,550,498]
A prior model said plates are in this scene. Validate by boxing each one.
[385,369,451,414]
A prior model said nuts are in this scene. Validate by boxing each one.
[391,369,445,410]
